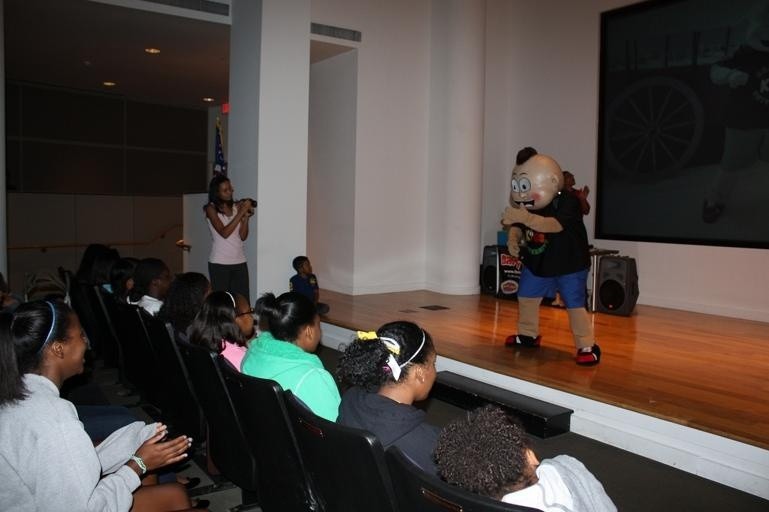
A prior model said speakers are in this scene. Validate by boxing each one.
[595,255,639,316]
[480,245,497,296]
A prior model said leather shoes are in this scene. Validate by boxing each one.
[182,477,200,490]
[189,498,210,509]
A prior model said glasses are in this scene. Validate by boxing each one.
[238,308,255,316]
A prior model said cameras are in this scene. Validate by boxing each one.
[239,198,257,208]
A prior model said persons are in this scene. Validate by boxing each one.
[561,170,591,216]
[502,145,604,366]
[77,243,257,376]
[434,404,620,511]
[334,320,449,480]
[289,255,330,316]
[240,289,343,424]
[202,176,256,298]
[0,298,197,510]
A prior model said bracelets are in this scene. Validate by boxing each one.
[130,455,146,474]
